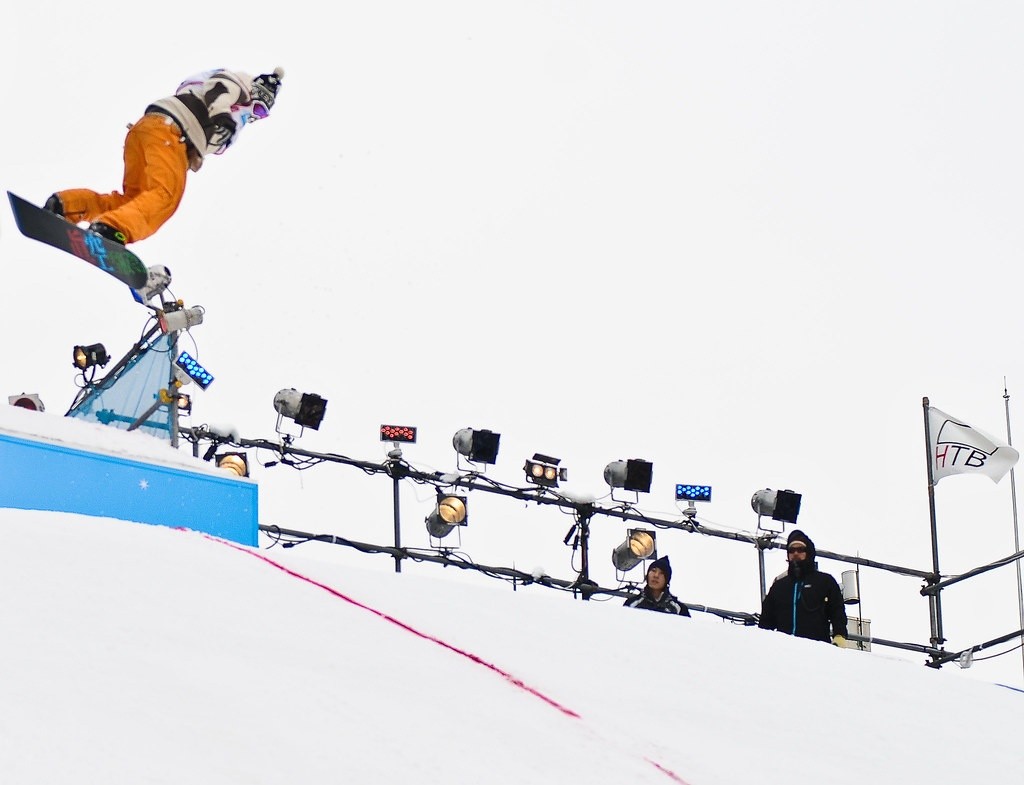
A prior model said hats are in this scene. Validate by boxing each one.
[249,68,285,111]
[788,534,807,547]
[647,555,672,584]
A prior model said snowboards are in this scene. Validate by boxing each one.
[5,189,148,291]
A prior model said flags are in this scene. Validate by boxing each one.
[927,407,1020,486]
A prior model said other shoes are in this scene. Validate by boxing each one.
[89,222,126,247]
[45,195,63,215]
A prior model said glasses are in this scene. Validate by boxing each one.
[250,100,269,120]
[788,547,805,554]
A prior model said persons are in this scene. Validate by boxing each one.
[622,554,694,619]
[757,529,851,650]
[39,65,286,247]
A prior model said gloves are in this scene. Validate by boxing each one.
[832,635,845,648]
[213,116,237,148]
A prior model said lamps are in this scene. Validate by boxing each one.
[214,451,250,481]
[613,527,659,585]
[9,391,44,412]
[273,387,329,438]
[156,304,207,336]
[603,458,656,504]
[522,453,569,490]
[171,394,195,415]
[453,428,502,476]
[379,424,418,453]
[674,484,713,517]
[171,351,215,392]
[72,342,115,391]
[426,492,470,540]
[752,487,802,534]
